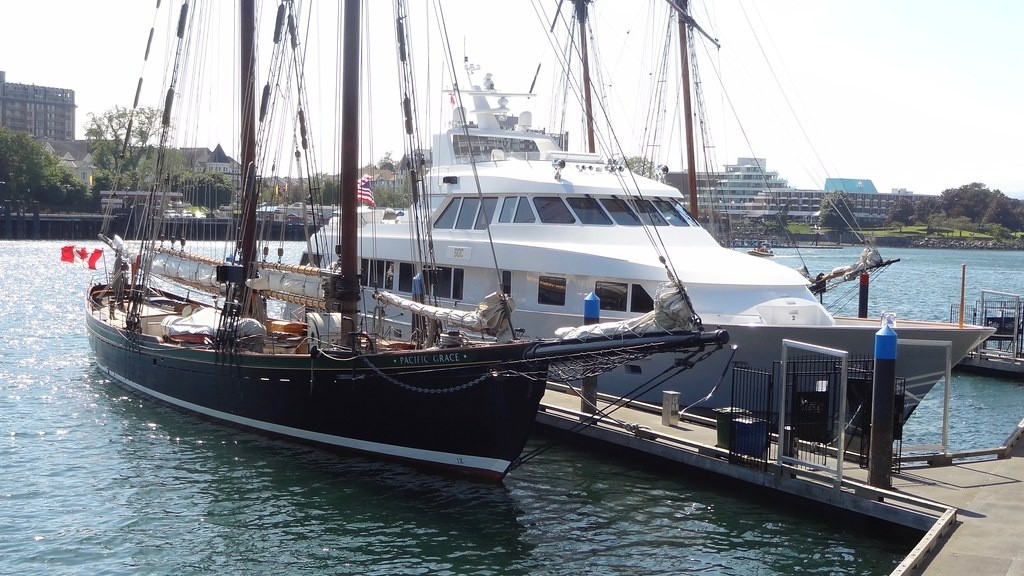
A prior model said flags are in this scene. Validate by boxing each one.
[357,173,376,211]
[59,245,105,269]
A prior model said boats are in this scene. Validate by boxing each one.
[84,231,552,484]
[299,60,995,455]
[747,245,775,257]
